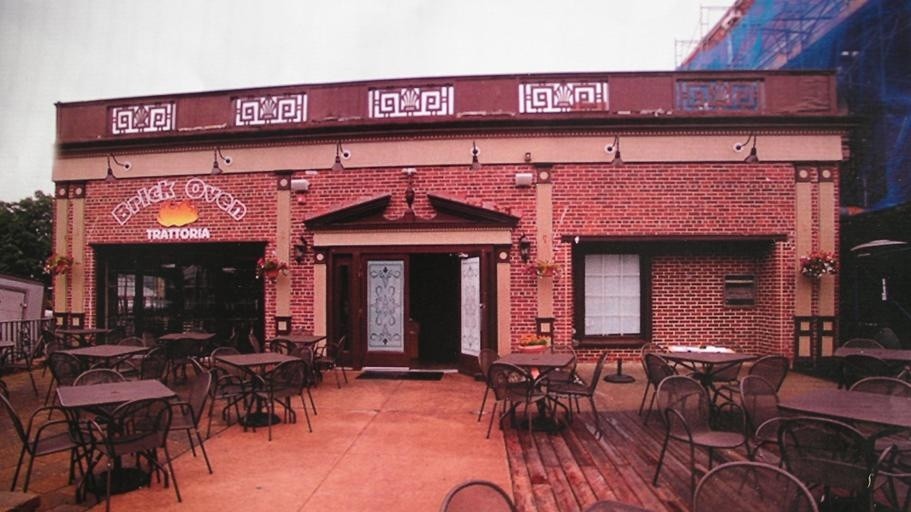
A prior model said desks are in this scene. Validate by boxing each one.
[56,380,177,501]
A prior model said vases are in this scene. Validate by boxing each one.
[546,267,553,280]
[270,269,277,278]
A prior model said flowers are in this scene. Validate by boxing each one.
[534,256,562,275]
[257,251,290,269]
[800,251,837,276]
[39,255,72,274]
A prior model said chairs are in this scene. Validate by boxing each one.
[475,343,609,440]
[3,318,348,440]
[0,393,96,495]
[75,370,135,458]
[153,370,214,477]
[91,396,181,511]
[640,338,910,510]
[441,479,516,511]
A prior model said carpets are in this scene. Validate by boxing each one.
[356,369,444,381]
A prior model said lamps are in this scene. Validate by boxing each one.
[294,236,310,264]
[518,234,533,263]
[331,139,350,173]
[212,147,233,177]
[604,135,623,167]
[514,172,534,186]
[105,153,132,182]
[469,142,484,171]
[732,131,762,164]
[290,179,307,189]
